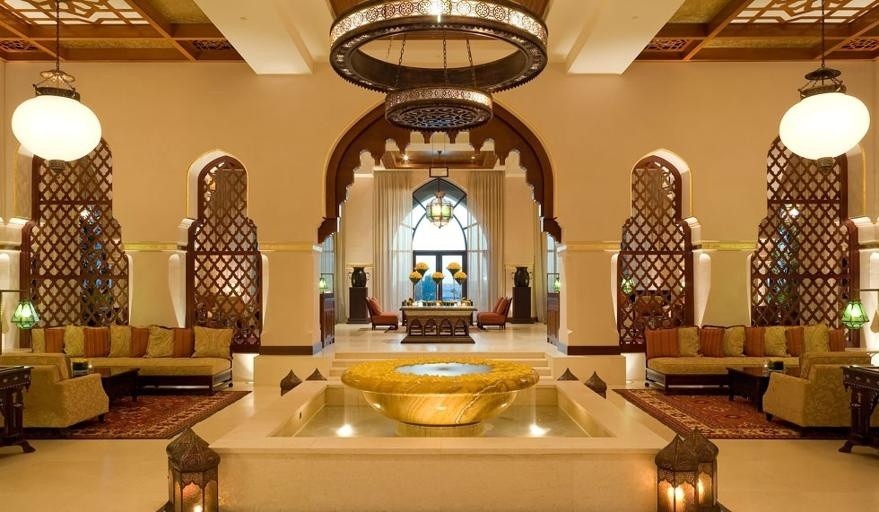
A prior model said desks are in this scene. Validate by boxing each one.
[0,366,36,453]
[839,363,879,454]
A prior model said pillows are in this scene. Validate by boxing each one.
[26,324,235,361]
[643,324,847,358]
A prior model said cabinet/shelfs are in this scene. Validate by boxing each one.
[547,293,560,345]
[320,294,335,348]
[345,288,370,325]
[511,287,533,325]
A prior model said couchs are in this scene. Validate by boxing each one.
[644,323,879,434]
[0,325,238,436]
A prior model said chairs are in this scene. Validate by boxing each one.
[364,295,512,338]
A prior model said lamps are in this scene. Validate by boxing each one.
[426,150,453,229]
[11,299,38,339]
[11,0,101,167]
[328,0,548,133]
[779,1,870,176]
[840,298,869,349]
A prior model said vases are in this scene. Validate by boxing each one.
[351,265,367,288]
[513,267,529,287]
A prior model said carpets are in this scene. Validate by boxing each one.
[0,385,253,440]
[612,386,850,440]
[400,334,475,344]
